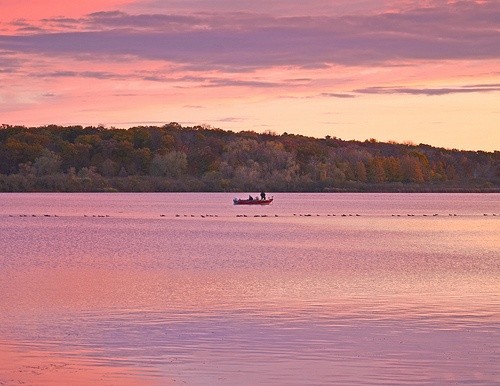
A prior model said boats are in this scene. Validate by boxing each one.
[232,196,273,205]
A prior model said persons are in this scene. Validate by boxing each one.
[248,195,254,200]
[260,191,266,200]
